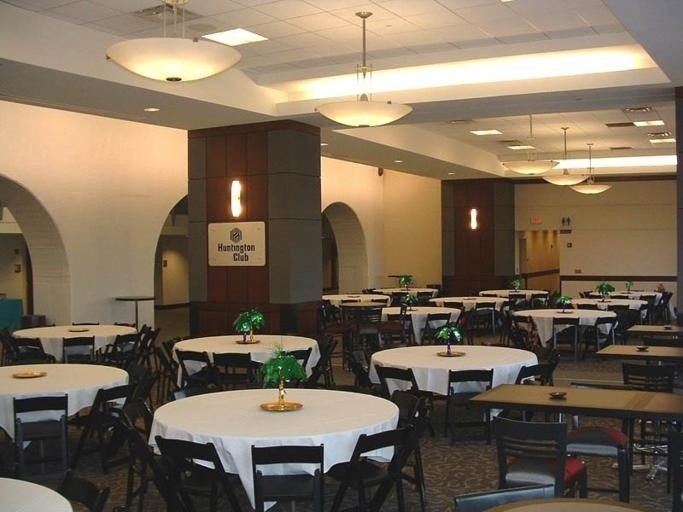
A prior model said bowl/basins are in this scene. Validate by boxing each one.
[635,346,647,350]
[664,326,671,329]
[549,391,567,399]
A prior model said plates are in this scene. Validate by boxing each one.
[70,329,91,333]
[348,295,360,298]
[12,370,48,378]
[464,297,476,300]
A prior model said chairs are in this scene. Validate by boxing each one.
[157,335,258,403]
[484,290,555,314]
[497,412,682,502]
[390,284,496,346]
[455,486,554,511]
[13,370,157,480]
[329,426,416,510]
[269,300,415,389]
[250,443,325,512]
[447,369,496,446]
[502,315,620,364]
[515,356,559,385]
[4,314,162,374]
[374,363,436,505]
[577,291,673,331]
[104,407,245,512]
[620,331,676,393]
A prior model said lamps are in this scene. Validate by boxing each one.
[101,1,242,88]
[497,115,614,196]
[311,9,417,128]
